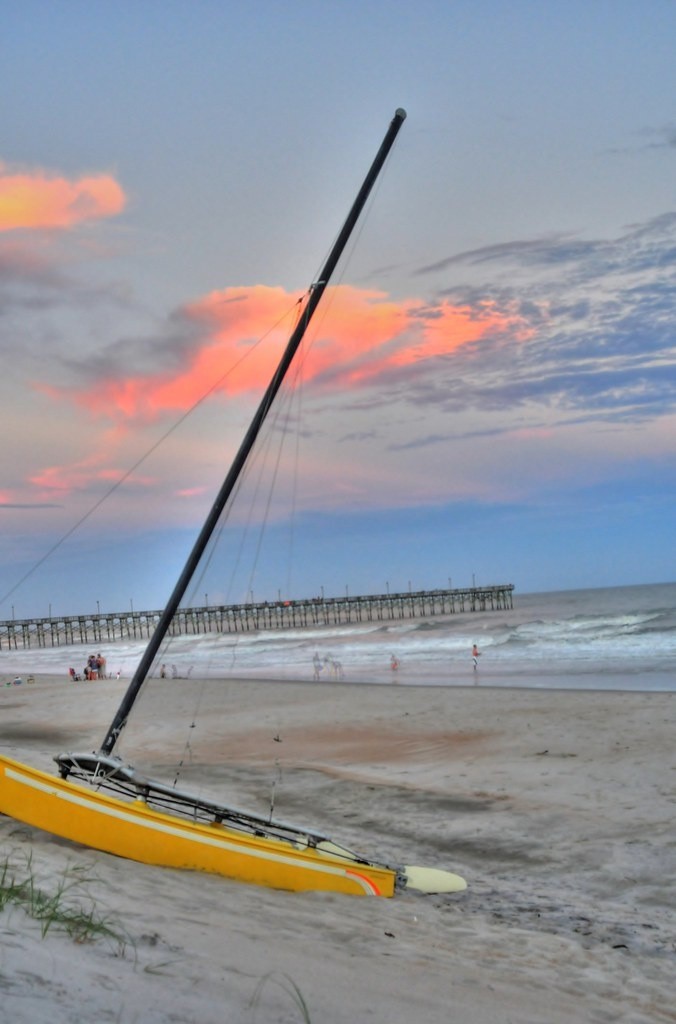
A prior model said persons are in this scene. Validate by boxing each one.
[391,653,399,671]
[12,676,36,685]
[159,663,194,679]
[310,651,346,682]
[472,644,481,672]
[67,654,121,681]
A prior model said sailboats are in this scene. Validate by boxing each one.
[0,99,415,899]
